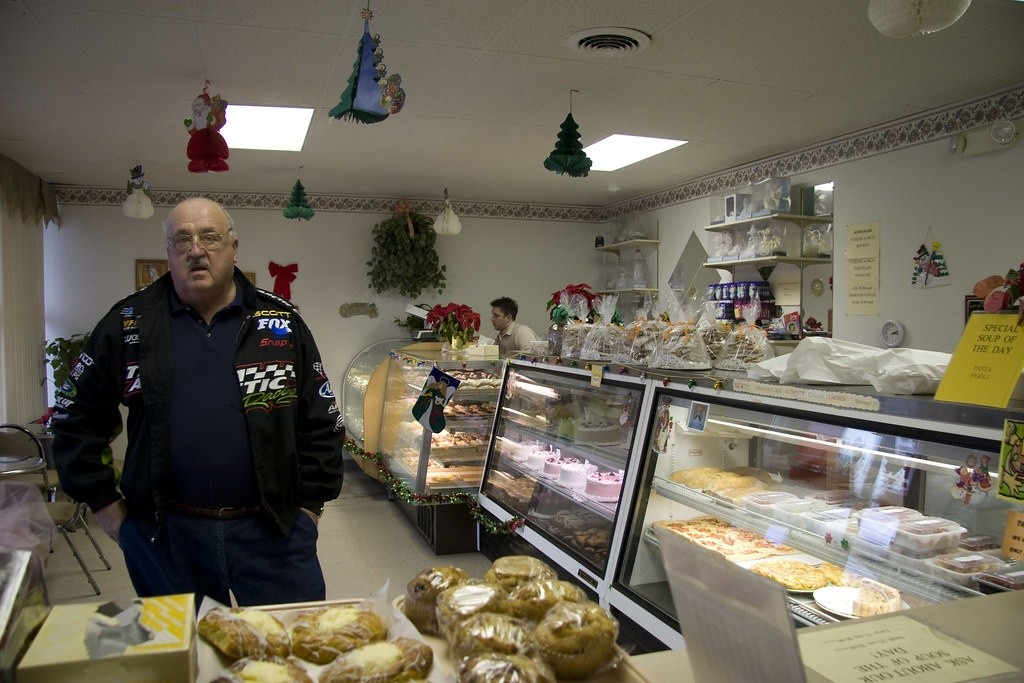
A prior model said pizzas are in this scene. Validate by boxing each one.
[657,515,801,562]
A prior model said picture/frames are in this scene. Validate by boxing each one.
[135,259,168,291]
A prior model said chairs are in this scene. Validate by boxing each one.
[0,424,112,596]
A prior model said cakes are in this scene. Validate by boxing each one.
[506,394,634,555]
[562,321,769,370]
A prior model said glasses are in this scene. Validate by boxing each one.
[166,229,234,255]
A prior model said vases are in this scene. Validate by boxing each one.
[452,336,459,348]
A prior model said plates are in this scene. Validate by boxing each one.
[734,552,911,619]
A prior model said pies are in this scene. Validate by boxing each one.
[852,577,900,617]
[752,559,862,589]
[667,465,779,500]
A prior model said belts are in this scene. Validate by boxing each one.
[161,499,263,520]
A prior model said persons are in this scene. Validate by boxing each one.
[490,296,540,356]
[183,95,230,173]
[48,198,346,619]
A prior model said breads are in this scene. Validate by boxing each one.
[400,368,503,482]
[198,555,432,683]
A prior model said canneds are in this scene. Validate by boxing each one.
[708,280,773,321]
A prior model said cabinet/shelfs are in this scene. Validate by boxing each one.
[339,339,1024,681]
[596,237,660,293]
[702,212,834,344]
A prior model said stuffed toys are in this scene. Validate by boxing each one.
[121,166,154,219]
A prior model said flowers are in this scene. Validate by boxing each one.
[426,302,481,348]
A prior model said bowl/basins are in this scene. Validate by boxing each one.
[528,341,547,355]
[924,548,1024,596]
[742,489,1002,571]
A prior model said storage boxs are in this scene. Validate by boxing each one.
[15,593,198,683]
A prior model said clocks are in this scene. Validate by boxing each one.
[880,320,904,348]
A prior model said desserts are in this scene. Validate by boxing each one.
[405,555,618,683]
